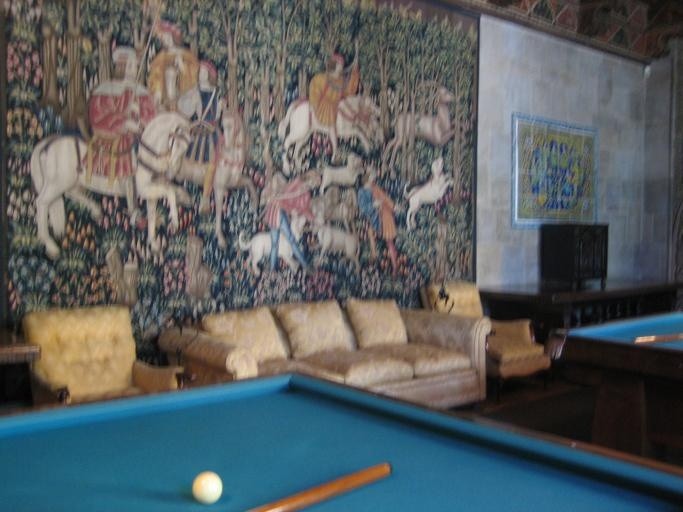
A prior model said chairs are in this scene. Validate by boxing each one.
[417,278,553,406]
[19,300,199,415]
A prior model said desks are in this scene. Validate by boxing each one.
[476,278,682,395]
[541,308,681,463]
[0,338,42,417]
[0,366,682,510]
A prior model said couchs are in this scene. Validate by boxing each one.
[156,292,496,414]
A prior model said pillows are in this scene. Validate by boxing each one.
[269,298,356,361]
[423,279,484,320]
[199,305,288,365]
[342,293,411,352]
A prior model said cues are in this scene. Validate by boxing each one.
[247,461,392,512]
[635,334,682,345]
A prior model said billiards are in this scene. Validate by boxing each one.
[194,472,223,503]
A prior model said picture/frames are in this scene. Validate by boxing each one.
[507,109,604,230]
